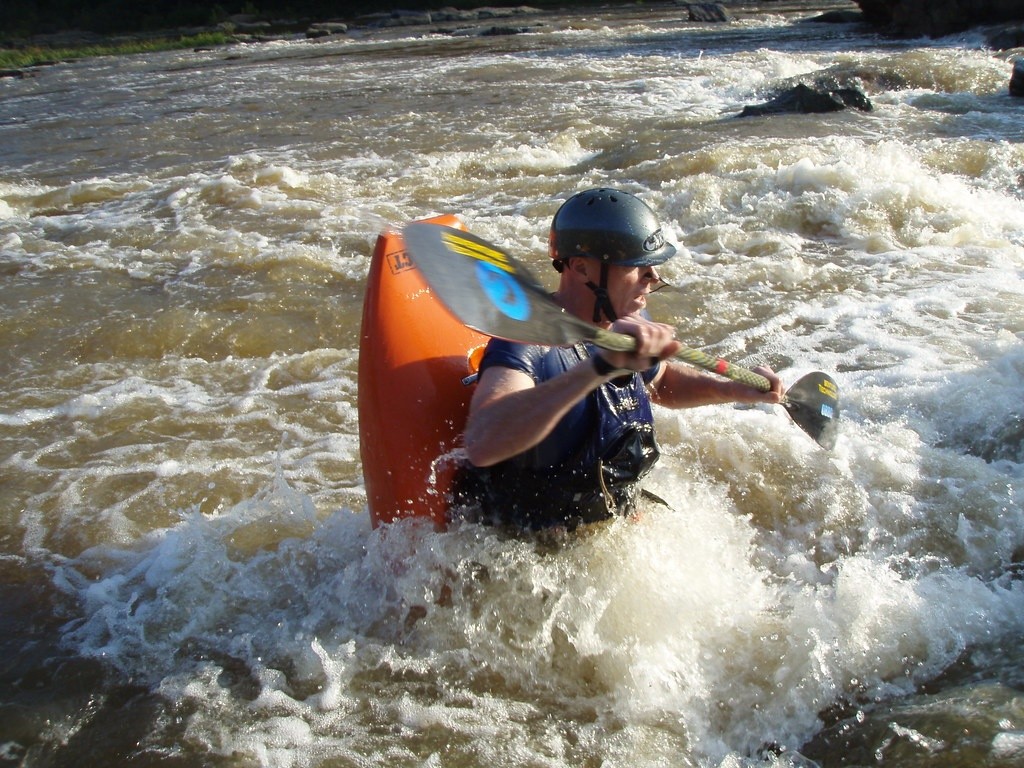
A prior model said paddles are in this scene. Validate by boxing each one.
[394,208,849,451]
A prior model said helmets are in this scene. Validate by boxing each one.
[548,187,676,268]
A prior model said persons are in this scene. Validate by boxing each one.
[448,188,785,531]
